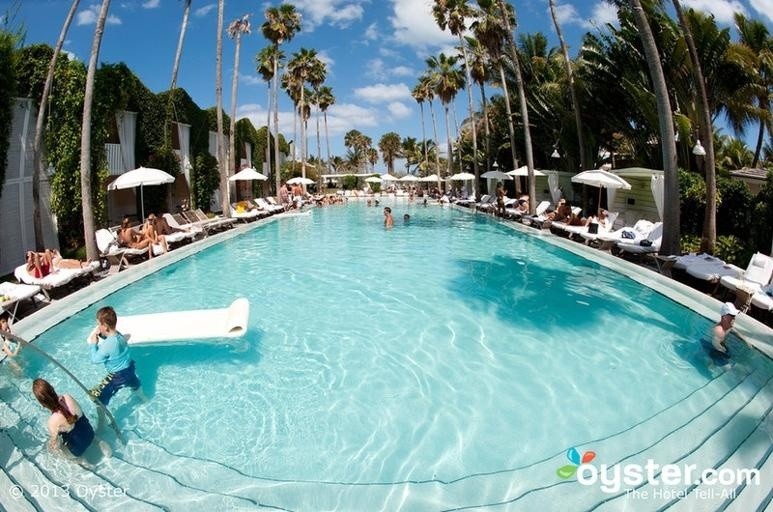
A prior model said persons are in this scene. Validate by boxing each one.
[30,377,112,469]
[24,174,609,281]
[702,301,755,371]
[0,318,27,377]
[86,304,146,432]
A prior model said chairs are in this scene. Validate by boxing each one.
[443,179,772,321]
[0,178,315,344]
[339,176,441,199]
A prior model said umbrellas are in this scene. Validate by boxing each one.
[505,165,546,196]
[226,167,267,199]
[568,166,632,217]
[106,165,177,224]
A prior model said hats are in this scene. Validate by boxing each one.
[721,302,740,318]
